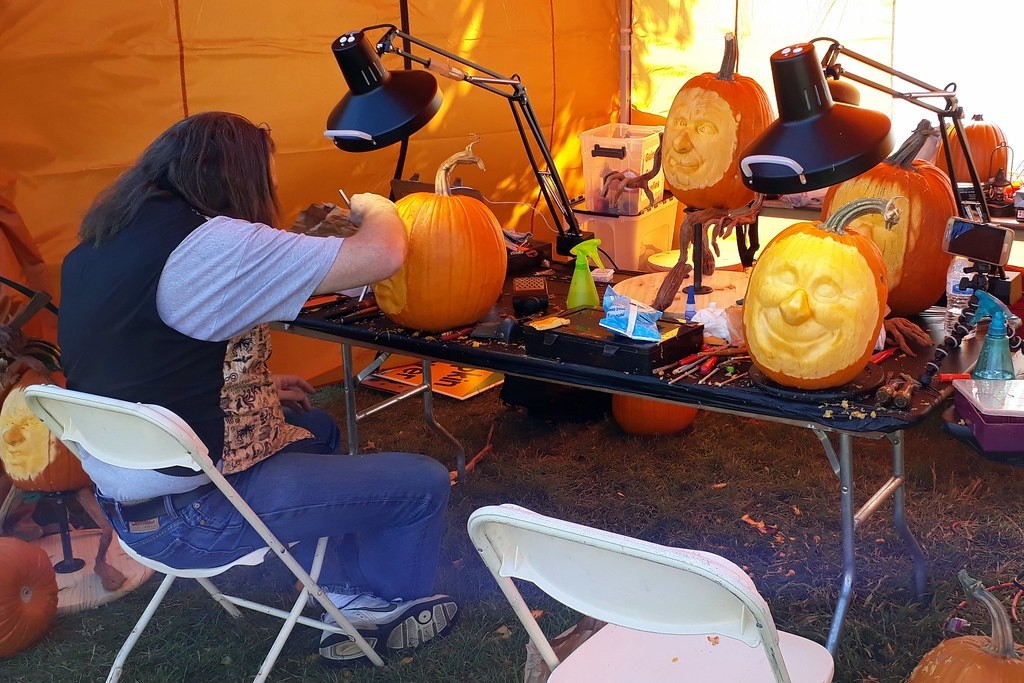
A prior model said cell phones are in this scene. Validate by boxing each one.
[941,216,1014,266]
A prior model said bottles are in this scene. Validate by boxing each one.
[945,255,977,340]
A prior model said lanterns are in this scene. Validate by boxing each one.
[661,32,773,211]
[818,119,961,316]
[1,366,93,492]
[742,197,901,390]
[369,139,508,331]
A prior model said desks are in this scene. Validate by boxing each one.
[270,237,934,680]
[684,196,1024,280]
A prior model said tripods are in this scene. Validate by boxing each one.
[918,261,1024,386]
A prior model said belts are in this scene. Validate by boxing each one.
[94,482,219,522]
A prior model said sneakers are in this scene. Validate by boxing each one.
[319,595,459,668]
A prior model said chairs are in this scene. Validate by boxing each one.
[21,384,386,683]
[468,501,836,681]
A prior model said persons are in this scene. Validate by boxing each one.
[57,110,462,663]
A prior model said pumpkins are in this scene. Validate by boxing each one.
[611,391,699,435]
[662,31,1008,389]
[370,151,507,329]
[904,568,1023,683]
[0,358,92,657]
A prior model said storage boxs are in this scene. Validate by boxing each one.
[567,189,679,274]
[577,121,666,216]
